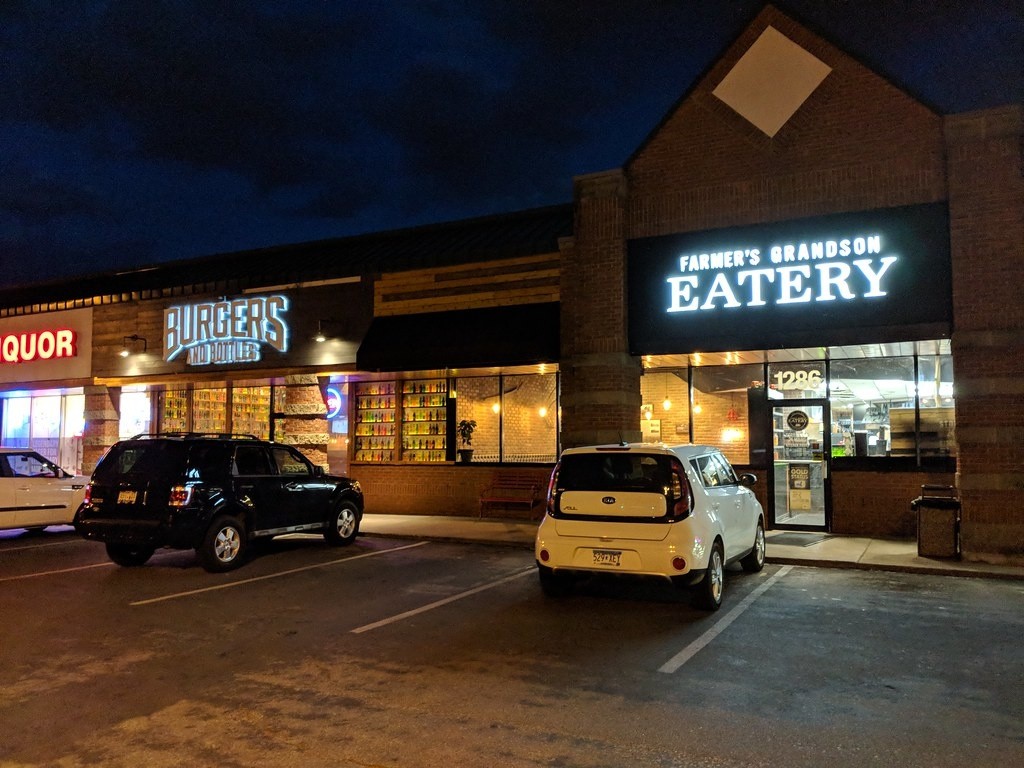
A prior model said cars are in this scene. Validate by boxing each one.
[0,447,90,531]
[535,431,766,612]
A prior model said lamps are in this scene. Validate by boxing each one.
[121,335,139,358]
[316,319,326,342]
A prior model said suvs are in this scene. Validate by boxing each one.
[73,433,365,573]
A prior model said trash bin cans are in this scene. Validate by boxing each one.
[910,483,962,559]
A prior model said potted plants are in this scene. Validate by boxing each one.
[456,419,477,462]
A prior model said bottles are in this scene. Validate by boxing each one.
[165,386,286,443]
[356,382,446,462]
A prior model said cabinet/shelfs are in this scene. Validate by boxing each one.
[402,391,446,461]
[772,407,785,460]
[356,394,395,461]
[164,386,287,443]
